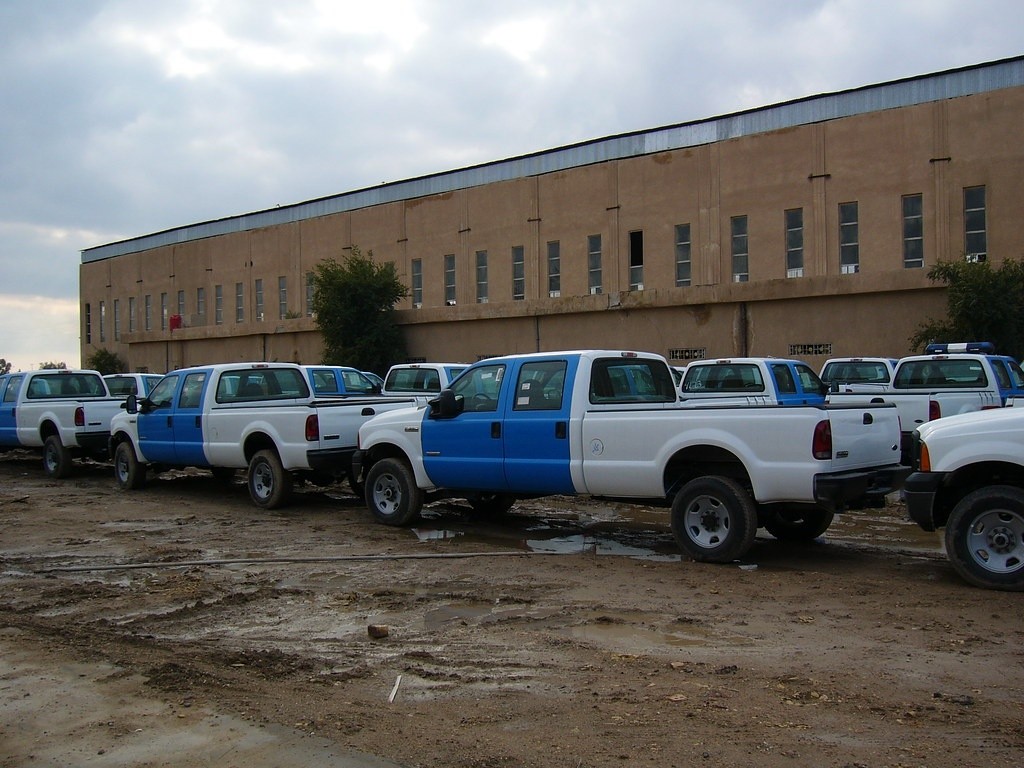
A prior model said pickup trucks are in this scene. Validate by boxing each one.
[81,340,1022,458]
[0,369,148,481]
[905,404,1024,596]
[106,357,417,508]
[346,347,911,568]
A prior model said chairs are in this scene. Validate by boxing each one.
[773,372,790,389]
[846,367,861,382]
[517,379,547,409]
[721,375,745,389]
[925,372,946,385]
[426,377,441,390]
[326,377,336,388]
[241,382,265,396]
